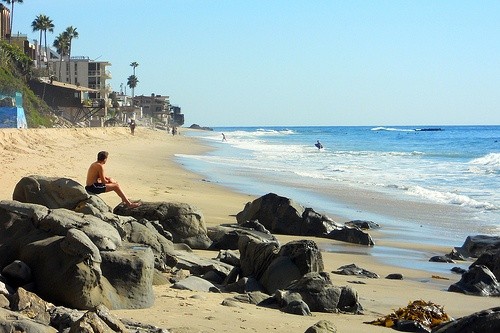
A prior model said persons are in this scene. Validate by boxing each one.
[85,151,142,209]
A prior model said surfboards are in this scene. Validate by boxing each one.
[315,143,323,148]
